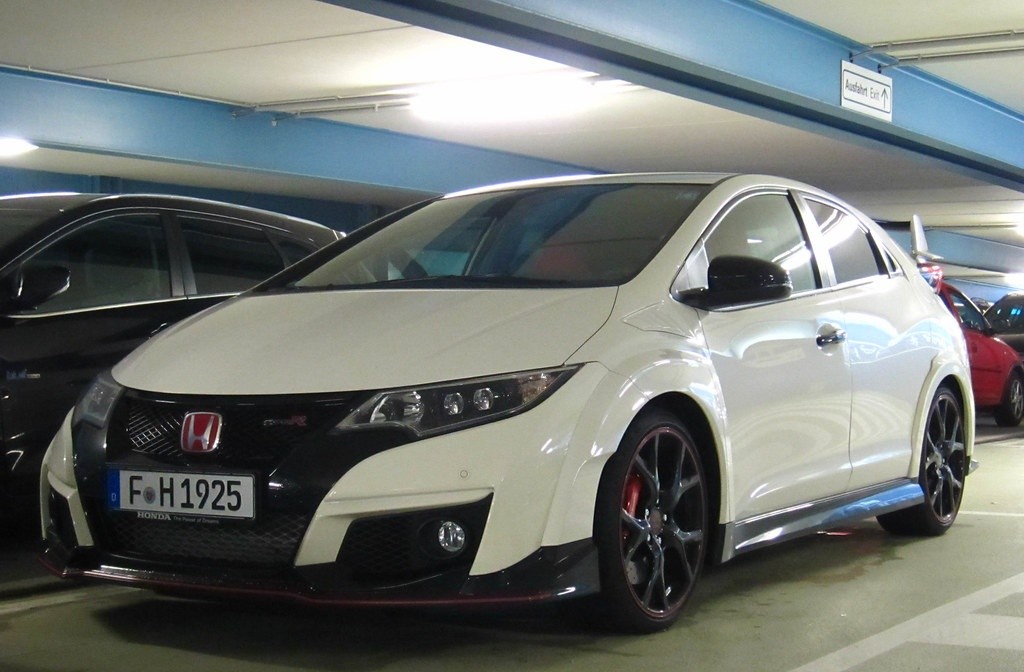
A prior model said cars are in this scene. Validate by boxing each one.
[958,289,1024,361]
[39,169,980,636]
[2,189,390,527]
[907,259,1024,430]
